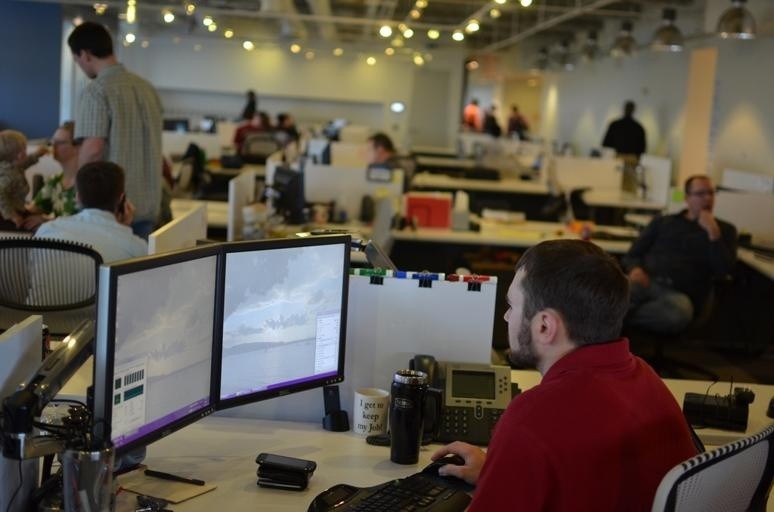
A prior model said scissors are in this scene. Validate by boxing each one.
[72,409,111,491]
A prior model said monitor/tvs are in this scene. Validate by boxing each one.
[160,112,346,227]
[216,234,352,410]
[90,244,219,463]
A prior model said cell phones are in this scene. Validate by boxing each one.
[120,194,126,214]
[255,453,316,473]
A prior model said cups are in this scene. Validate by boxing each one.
[352,388,390,438]
[390,369,443,466]
[62,449,110,511]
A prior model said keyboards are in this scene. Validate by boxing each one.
[307,473,472,512]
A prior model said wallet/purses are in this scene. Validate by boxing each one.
[257,464,313,490]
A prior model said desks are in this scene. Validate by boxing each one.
[0,117,773,510]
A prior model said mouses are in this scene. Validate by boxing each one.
[421,457,465,477]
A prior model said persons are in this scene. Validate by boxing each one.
[482,104,502,136]
[23,121,85,233]
[274,113,298,140]
[462,98,482,134]
[173,143,212,199]
[507,103,529,139]
[366,134,408,194]
[160,154,173,191]
[617,175,738,382]
[239,92,255,124]
[68,22,164,243]
[23,159,149,334]
[0,128,48,234]
[234,112,273,164]
[602,99,647,195]
[429,236,701,511]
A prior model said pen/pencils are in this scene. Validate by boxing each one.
[144,470,205,486]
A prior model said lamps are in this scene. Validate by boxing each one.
[524,1,759,76]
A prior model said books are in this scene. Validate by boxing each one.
[112,468,218,505]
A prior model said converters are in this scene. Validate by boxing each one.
[681,391,749,433]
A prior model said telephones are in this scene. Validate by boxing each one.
[409,354,511,446]
